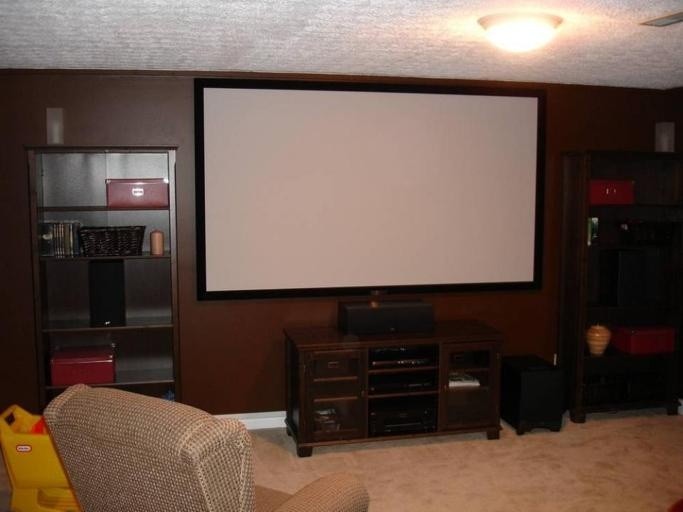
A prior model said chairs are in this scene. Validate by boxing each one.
[41,382,371,512]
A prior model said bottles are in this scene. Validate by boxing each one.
[586,325,612,355]
[150,229,164,255]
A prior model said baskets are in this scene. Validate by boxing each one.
[78,224,146,257]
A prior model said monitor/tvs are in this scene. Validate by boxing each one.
[194,78,545,301]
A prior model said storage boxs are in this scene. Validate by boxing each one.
[105,178,169,209]
[49,345,117,386]
[589,178,636,206]
[613,325,676,355]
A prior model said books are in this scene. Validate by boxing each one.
[446,372,481,388]
[37,220,84,258]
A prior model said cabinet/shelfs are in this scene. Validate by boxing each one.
[555,147,683,423]
[24,143,183,415]
[500,354,562,435]
[282,319,503,457]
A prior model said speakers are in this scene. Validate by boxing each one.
[340,301,433,335]
[89,259,125,327]
[599,248,646,305]
